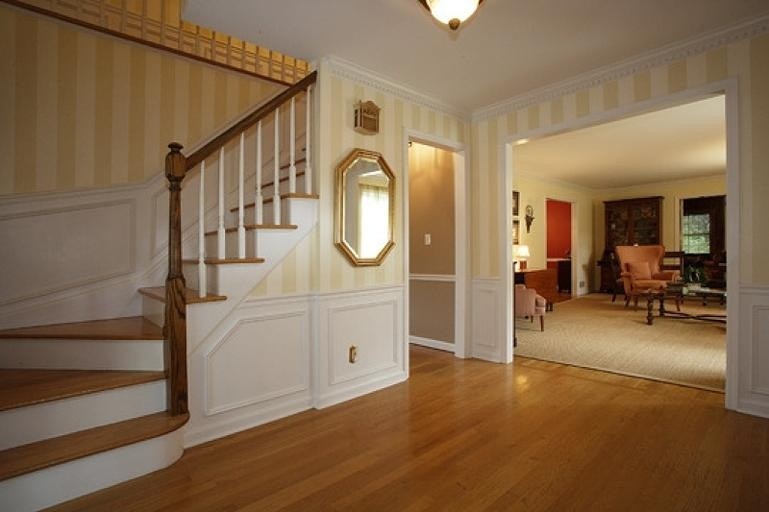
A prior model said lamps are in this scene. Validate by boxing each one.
[418,1,483,31]
[516,245,531,269]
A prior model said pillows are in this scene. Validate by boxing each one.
[627,259,652,280]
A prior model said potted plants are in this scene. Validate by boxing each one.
[681,265,705,292]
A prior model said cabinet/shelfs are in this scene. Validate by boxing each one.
[514,269,557,312]
[596,195,665,294]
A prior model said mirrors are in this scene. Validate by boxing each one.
[333,147,397,268]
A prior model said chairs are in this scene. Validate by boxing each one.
[514,284,547,332]
[609,252,627,303]
[614,245,681,312]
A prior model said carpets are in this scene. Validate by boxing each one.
[512,294,728,395]
